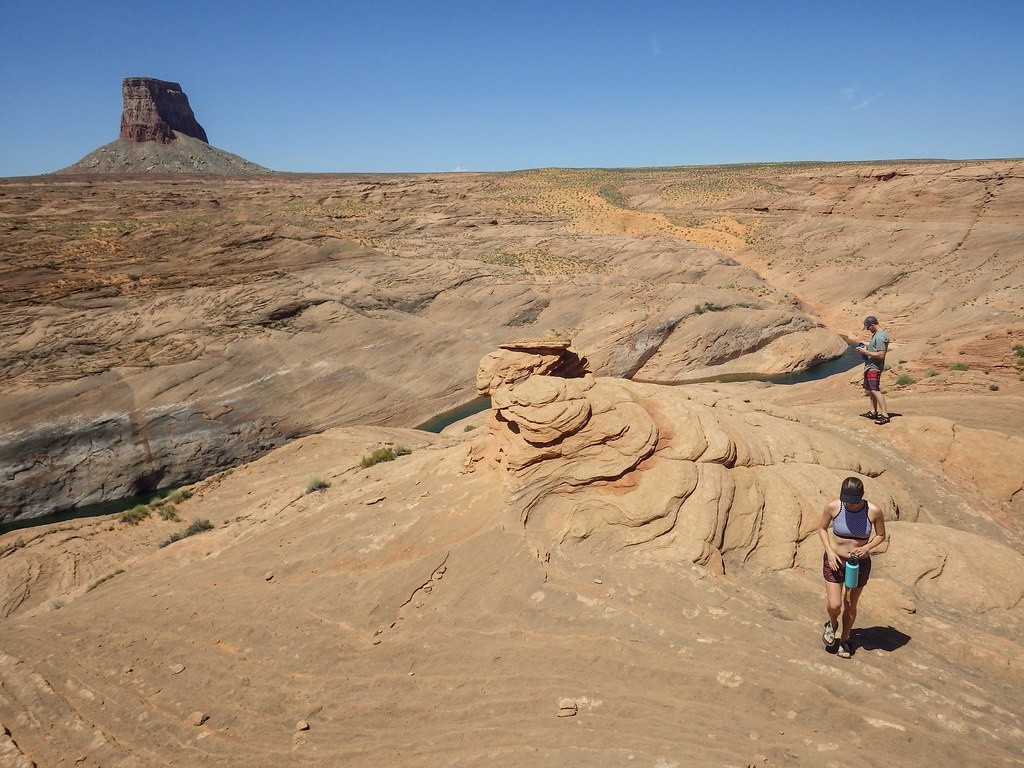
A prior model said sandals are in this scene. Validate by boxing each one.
[860,411,878,419]
[822,620,838,647]
[874,415,890,425]
[837,640,851,659]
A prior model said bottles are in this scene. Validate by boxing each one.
[845,556,859,588]
[859,343,868,360]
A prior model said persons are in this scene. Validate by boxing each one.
[858,316,891,424]
[819,476,885,658]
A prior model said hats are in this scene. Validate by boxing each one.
[862,316,877,330]
[840,488,864,504]
